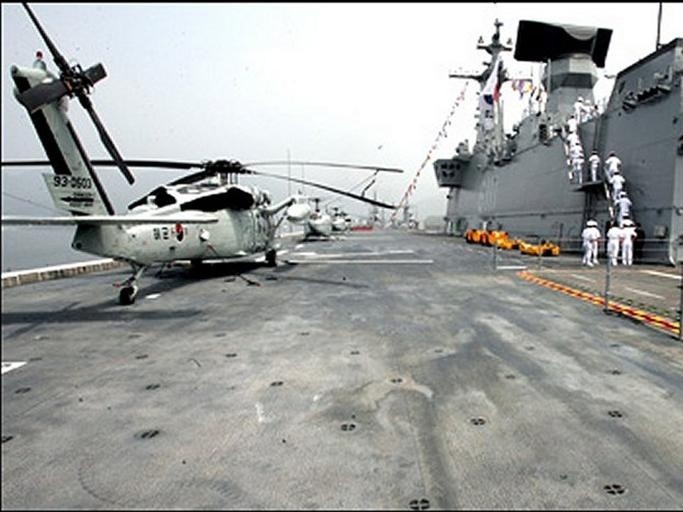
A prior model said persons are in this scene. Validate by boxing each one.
[580,221,594,267]
[620,220,638,266]
[34,51,46,73]
[564,96,635,228]
[607,221,622,266]
[591,220,601,265]
